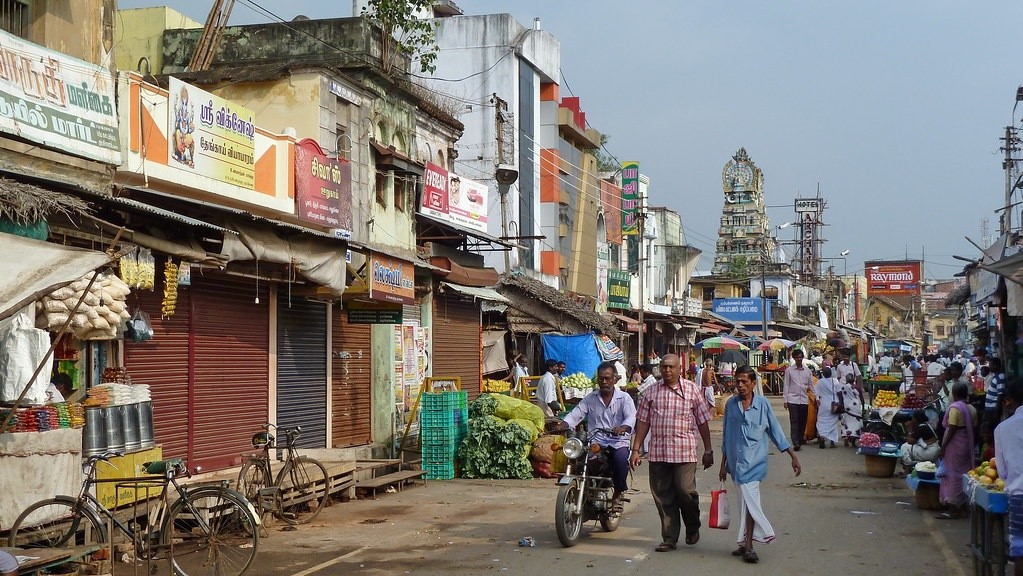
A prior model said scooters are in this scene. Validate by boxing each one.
[860,398,944,451]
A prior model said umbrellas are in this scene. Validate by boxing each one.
[694,335,750,368]
[717,350,746,385]
[746,335,766,344]
[756,339,795,351]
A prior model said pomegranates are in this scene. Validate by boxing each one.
[967,459,1004,490]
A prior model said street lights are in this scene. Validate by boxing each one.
[999,84,1023,239]
[759,221,791,365]
[829,266,879,329]
[496,163,517,282]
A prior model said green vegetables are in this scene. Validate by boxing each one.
[457,392,534,479]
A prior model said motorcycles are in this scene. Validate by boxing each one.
[549,424,630,547]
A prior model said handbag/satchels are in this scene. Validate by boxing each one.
[709,479,730,529]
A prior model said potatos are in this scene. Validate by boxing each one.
[901,393,924,408]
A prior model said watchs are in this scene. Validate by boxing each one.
[704,450,713,454]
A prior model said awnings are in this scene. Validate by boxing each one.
[776,322,885,339]
[614,316,646,333]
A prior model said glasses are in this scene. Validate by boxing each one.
[597,376,614,382]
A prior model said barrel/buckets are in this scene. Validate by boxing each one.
[81,401,154,458]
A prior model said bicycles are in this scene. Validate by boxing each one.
[237,424,329,533]
[6,452,263,576]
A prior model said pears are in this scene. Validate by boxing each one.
[561,371,592,388]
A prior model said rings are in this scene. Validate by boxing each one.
[709,464,712,466]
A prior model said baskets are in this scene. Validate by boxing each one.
[881,442,899,453]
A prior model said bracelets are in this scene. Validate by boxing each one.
[632,449,639,453]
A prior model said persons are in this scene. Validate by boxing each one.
[550,362,637,512]
[629,354,713,552]
[719,366,800,562]
[994,378,1023,576]
[500,342,1007,459]
[935,383,977,520]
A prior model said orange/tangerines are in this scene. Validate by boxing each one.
[874,390,899,407]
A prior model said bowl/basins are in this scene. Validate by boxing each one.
[857,446,881,454]
[916,470,936,480]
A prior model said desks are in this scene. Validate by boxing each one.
[856,447,902,477]
[905,474,940,509]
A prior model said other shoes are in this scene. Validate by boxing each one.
[830,444,837,448]
[899,471,910,478]
[936,512,955,519]
[732,547,746,555]
[686,530,699,544]
[744,549,758,561]
[656,542,676,551]
[801,441,805,444]
[820,444,824,448]
[794,444,800,451]
[831,401,843,413]
[844,443,857,448]
[612,497,624,512]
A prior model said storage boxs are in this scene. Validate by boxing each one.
[422,391,469,480]
